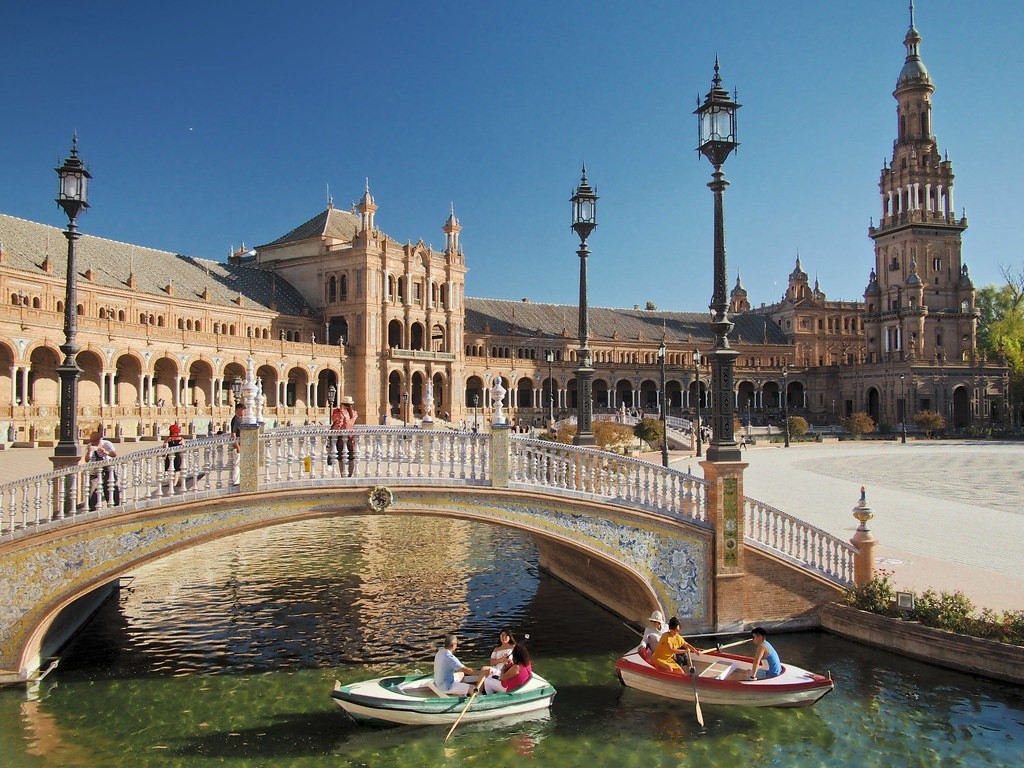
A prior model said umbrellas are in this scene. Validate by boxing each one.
[445,411,449,417]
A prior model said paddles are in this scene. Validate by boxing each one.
[444,673,488,743]
[700,638,754,654]
[687,648,705,727]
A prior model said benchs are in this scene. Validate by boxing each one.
[698,660,737,680]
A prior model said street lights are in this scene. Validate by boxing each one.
[693,348,702,457]
[569,160,596,445]
[402,391,409,439]
[783,371,790,447]
[473,394,479,432]
[48,129,93,517]
[657,342,669,467]
[327,385,336,424]
[231,377,242,411]
[692,53,742,463]
[900,374,906,443]
[547,350,556,433]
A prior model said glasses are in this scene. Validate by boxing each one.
[650,621,658,623]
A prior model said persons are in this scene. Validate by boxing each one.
[84,432,119,512]
[740,435,747,450]
[434,635,490,698]
[690,426,710,443]
[640,611,689,667]
[231,403,247,485]
[616,407,645,421]
[484,644,531,695]
[652,617,699,674]
[329,396,358,479]
[209,428,213,437]
[476,629,517,693]
[746,627,781,680]
[217,428,223,435]
[436,408,558,439]
[163,425,185,487]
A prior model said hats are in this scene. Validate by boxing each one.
[340,396,355,404]
[445,635,459,645]
[649,611,664,623]
[170,425,179,433]
[236,404,247,409]
[90,432,101,446]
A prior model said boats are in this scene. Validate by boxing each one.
[329,668,557,726]
[615,643,834,708]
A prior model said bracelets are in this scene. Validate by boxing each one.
[87,453,90,454]
[750,676,755,679]
